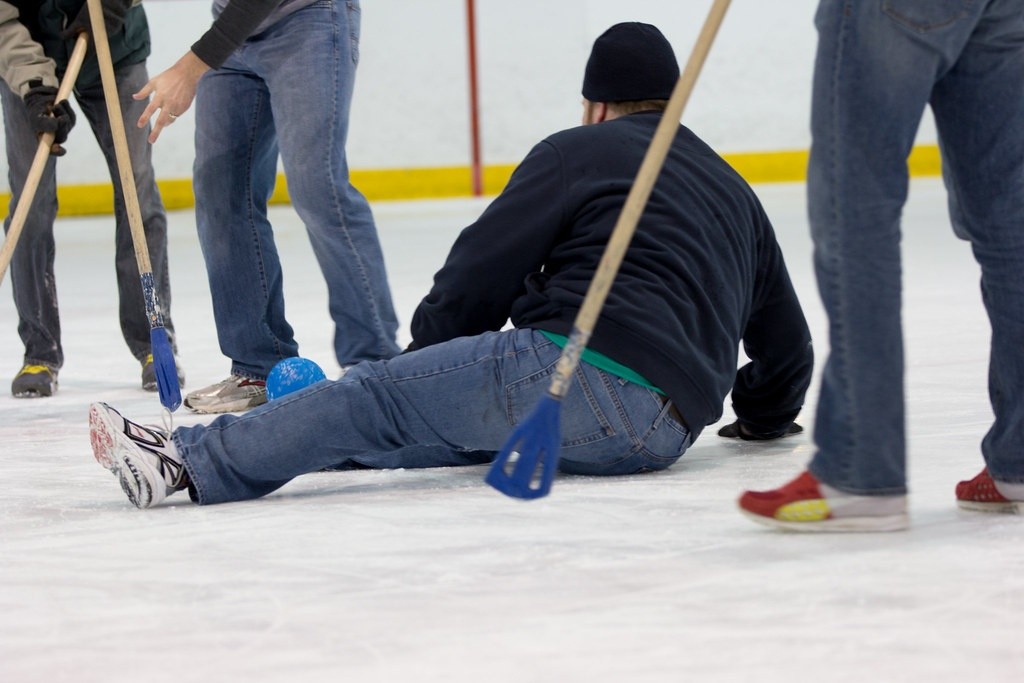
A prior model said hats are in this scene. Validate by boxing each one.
[580,21,680,103]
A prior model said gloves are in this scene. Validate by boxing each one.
[58,0,131,42]
[720,417,804,441]
[22,86,77,159]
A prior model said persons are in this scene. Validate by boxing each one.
[87,19,814,511]
[132,0,400,413]
[739,0,1024,535]
[0,0,185,398]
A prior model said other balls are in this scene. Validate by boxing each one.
[266,356,329,403]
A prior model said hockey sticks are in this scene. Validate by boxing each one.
[72,2,184,414]
[1,30,90,291]
[486,2,746,504]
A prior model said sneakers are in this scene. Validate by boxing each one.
[88,401,192,508]
[13,362,57,398]
[739,468,908,531]
[954,464,1022,516]
[139,352,185,389]
[183,375,270,414]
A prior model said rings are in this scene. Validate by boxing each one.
[169,113,179,119]
